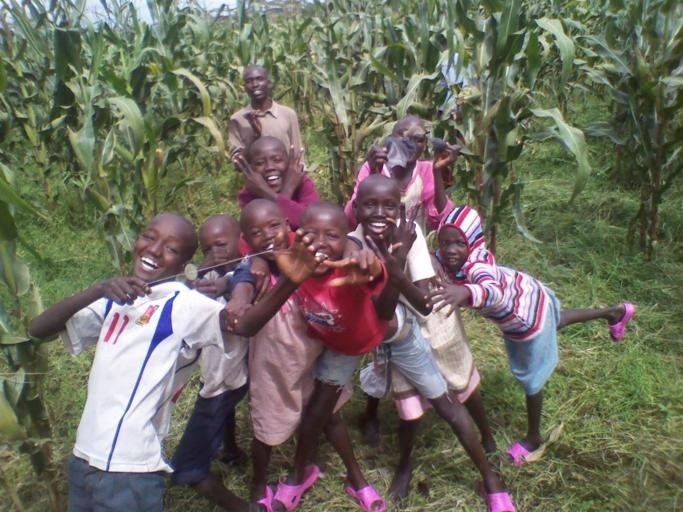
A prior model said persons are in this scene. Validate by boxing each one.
[30,211,329,512]
[171,66,515,512]
[424,206,634,464]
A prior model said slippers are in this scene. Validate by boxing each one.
[480,482,517,511]
[608,302,634,341]
[256,485,274,511]
[272,465,320,511]
[509,440,542,464]
[344,480,387,512]
